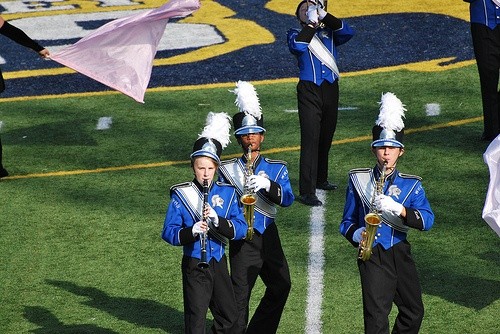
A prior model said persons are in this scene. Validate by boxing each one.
[162,150,249,334]
[287,0,354,206]
[0,15,50,178]
[340,134,435,334]
[462,0,500,141]
[218,121,295,334]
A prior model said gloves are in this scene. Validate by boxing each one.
[317,5,327,20]
[374,194,403,217]
[249,175,270,193]
[353,227,367,243]
[306,5,318,26]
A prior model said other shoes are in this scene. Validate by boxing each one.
[0,164,8,176]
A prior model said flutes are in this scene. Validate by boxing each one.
[197,179,210,271]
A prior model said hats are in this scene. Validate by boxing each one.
[296,0,325,13]
[371,92,405,149]
[189,112,230,165]
[233,81,266,138]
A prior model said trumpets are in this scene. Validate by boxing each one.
[307,0,325,29]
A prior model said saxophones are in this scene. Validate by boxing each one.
[240,143,259,241]
[359,159,388,261]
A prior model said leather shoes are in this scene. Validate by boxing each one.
[299,194,322,206]
[316,183,338,190]
